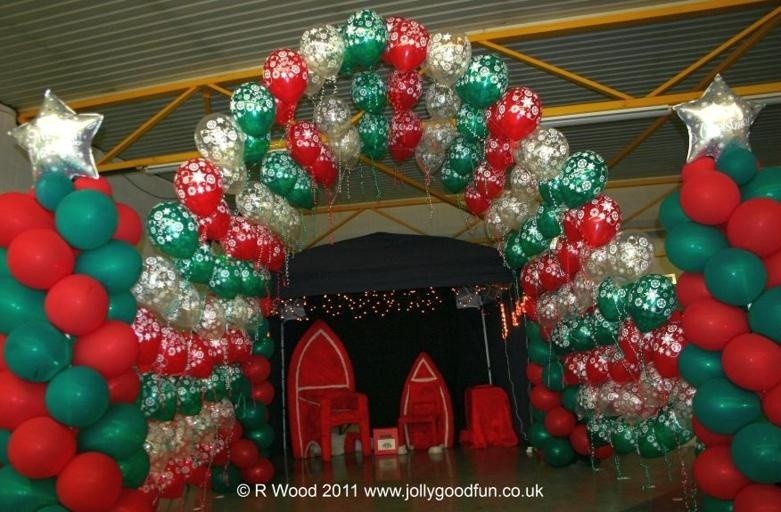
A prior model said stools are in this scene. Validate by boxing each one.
[462,384,518,452]
[372,425,399,457]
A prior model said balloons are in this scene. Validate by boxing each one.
[1,9,781,512]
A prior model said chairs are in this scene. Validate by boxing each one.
[288,320,372,465]
[398,352,454,454]
